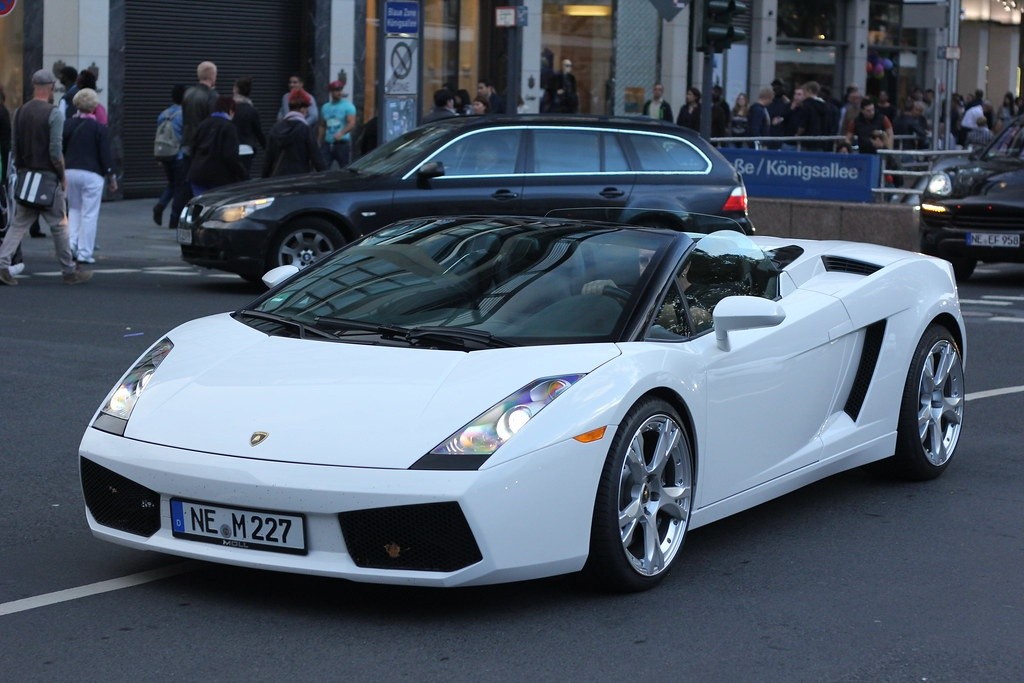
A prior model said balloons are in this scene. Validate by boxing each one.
[867,55,893,80]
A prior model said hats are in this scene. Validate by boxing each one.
[329,80,344,90]
[32,69,55,83]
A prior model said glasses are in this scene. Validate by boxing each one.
[639,256,649,268]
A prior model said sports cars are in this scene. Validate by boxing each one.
[77,205,967,591]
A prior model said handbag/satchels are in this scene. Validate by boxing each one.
[14,169,58,208]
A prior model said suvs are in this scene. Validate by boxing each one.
[917,116,1024,283]
[177,115,756,277]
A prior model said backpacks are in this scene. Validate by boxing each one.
[154,110,184,167]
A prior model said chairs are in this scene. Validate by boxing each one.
[687,229,776,323]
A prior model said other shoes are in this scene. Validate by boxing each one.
[62,270,93,284]
[153,205,162,225]
[31,230,47,239]
[169,214,180,229]
[0,269,18,286]
[8,263,25,276]
[77,253,95,263]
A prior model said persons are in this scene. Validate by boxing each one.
[580,230,731,334]
[264,87,328,177]
[277,75,318,127]
[642,78,1024,188]
[1,66,122,290]
[545,58,578,114]
[317,81,357,169]
[149,60,261,231]
[422,80,503,121]
